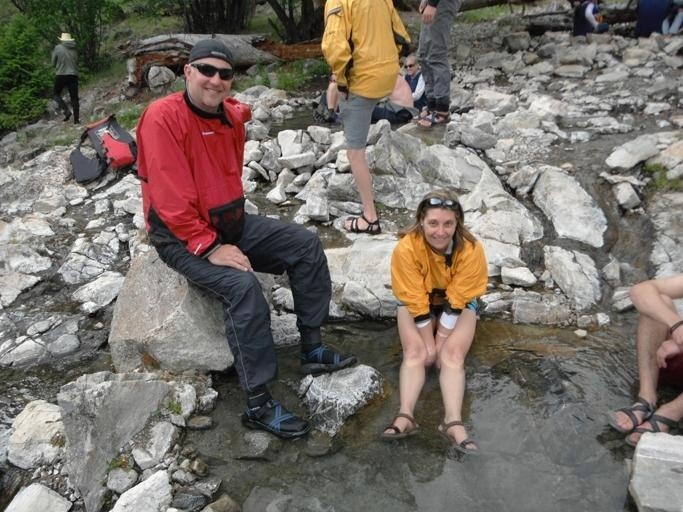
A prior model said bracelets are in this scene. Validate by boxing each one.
[434,330,448,338]
[665,317,683,334]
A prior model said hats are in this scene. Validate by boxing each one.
[56,32,75,41]
[190,38,235,68]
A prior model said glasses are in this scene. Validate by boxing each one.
[189,63,234,81]
[404,64,414,68]
[428,197,455,208]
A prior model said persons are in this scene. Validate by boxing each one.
[321,1,412,237]
[661,1,683,36]
[376,188,490,457]
[369,68,415,127]
[413,1,464,132]
[605,273,683,446]
[48,33,81,125]
[400,54,428,114]
[132,37,358,440]
[322,66,344,121]
[570,1,607,34]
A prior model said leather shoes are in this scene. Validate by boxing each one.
[74,120,79,124]
[63,112,72,121]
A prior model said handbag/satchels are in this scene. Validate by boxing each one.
[69,149,103,184]
[81,114,138,170]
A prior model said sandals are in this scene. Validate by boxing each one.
[437,419,479,455]
[376,412,420,440]
[345,213,381,235]
[242,400,312,437]
[413,107,451,129]
[300,344,357,376]
[607,396,680,450]
